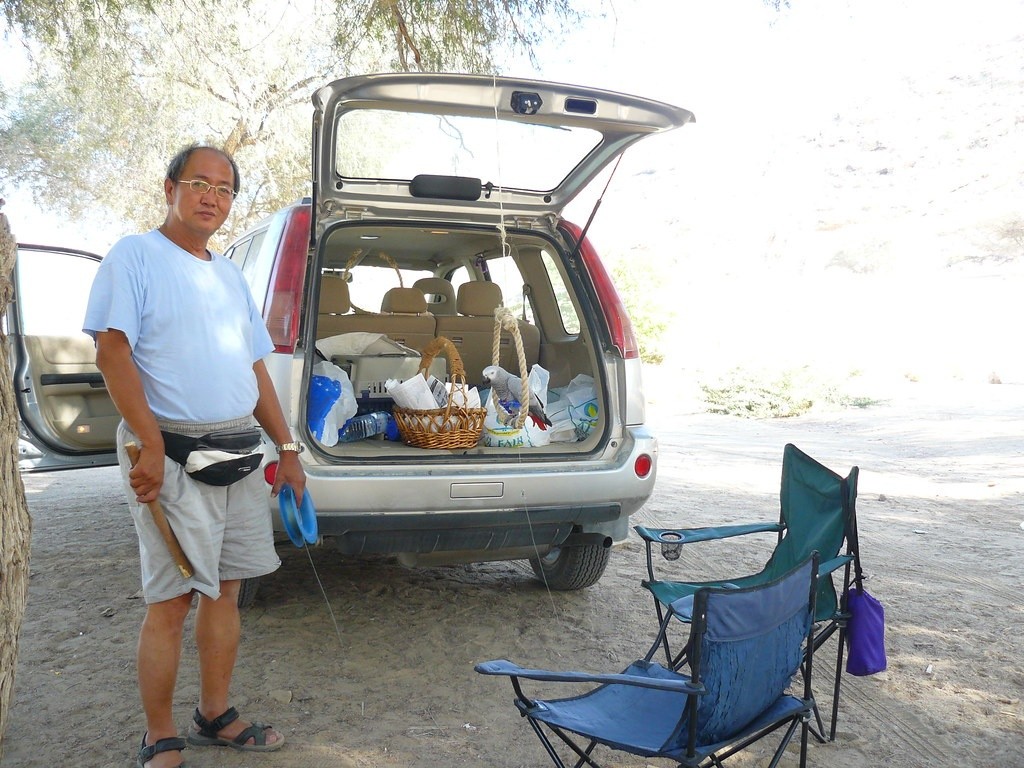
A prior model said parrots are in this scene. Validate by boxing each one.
[481,365,553,431]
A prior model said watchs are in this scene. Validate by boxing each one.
[275,440,301,454]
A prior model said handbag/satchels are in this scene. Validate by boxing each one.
[838,585,886,677]
[120,419,265,486]
[307,360,359,449]
[482,363,599,449]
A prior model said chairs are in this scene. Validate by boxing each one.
[473,548,820,767]
[633,442,860,746]
[434,281,540,386]
[413,277,456,316]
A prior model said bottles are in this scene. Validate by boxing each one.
[337,409,392,443]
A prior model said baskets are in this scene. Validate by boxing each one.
[391,336,487,449]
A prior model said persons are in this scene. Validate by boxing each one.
[82,146,307,768]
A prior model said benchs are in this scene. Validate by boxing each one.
[316,276,437,369]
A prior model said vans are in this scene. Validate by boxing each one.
[5,68,699,609]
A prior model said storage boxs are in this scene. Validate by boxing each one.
[349,356,449,418]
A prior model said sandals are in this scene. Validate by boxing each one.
[187,706,286,752]
[136,732,186,768]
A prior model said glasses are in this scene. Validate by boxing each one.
[175,180,237,199]
